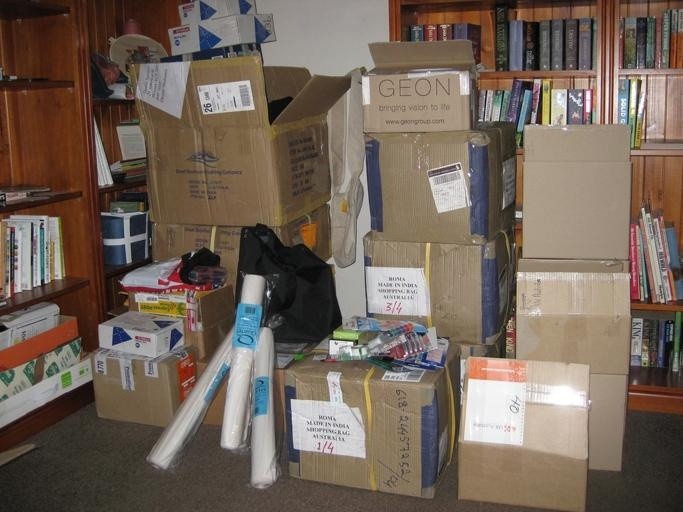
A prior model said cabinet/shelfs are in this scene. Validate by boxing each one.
[79,2,180,199]
[0,1,100,448]
[513,1,607,294]
[603,2,681,414]
[390,0,511,121]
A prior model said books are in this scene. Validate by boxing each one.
[94,117,149,190]
[411,23,481,67]
[477,78,593,145]
[628,204,683,303]
[495,4,593,71]
[0,214,66,306]
[619,8,683,70]
[631,312,683,371]
[619,79,646,147]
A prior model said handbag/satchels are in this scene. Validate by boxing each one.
[233,223,342,342]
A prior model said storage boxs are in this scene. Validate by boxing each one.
[521,121,628,259]
[458,357,590,508]
[93,346,194,427]
[125,284,235,331]
[367,125,516,243]
[178,0,258,24]
[361,230,507,346]
[285,336,460,498]
[169,13,277,55]
[104,212,149,270]
[198,341,308,433]
[127,58,352,227]
[185,316,234,357]
[591,375,628,475]
[150,205,329,283]
[516,258,630,376]
[363,44,477,132]
[98,310,183,356]
